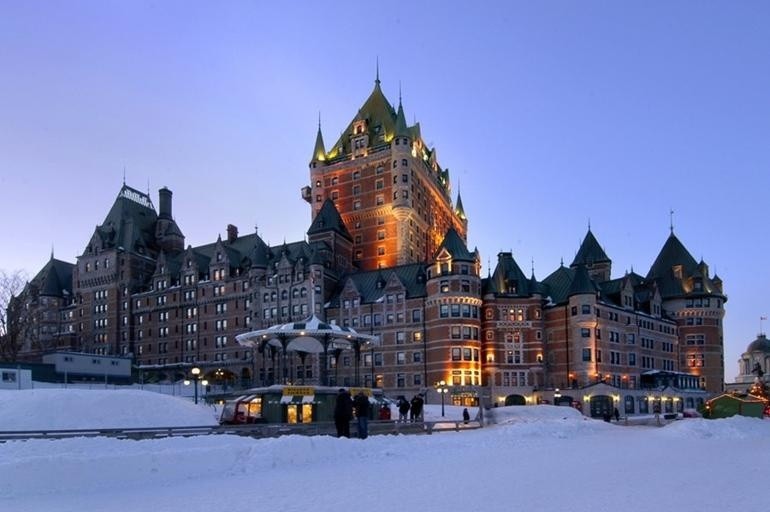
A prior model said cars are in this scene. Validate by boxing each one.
[683,411,703,418]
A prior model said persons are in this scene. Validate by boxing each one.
[462,407,470,425]
[614,407,621,421]
[396,395,411,422]
[378,401,391,419]
[603,408,611,423]
[333,387,354,439]
[410,393,423,422]
[352,391,371,438]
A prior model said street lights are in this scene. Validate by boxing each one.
[183,368,207,405]
[437,379,448,417]
[216,368,224,384]
[554,388,562,406]
